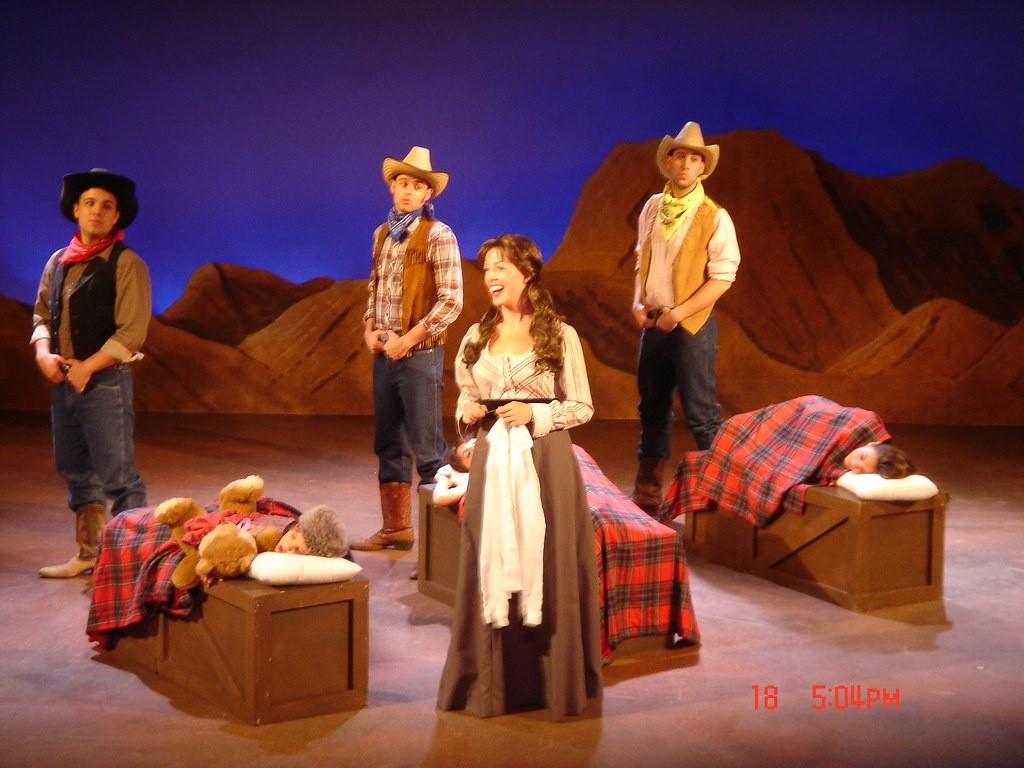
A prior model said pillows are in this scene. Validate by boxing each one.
[434,463,469,488]
[835,471,938,502]
[248,552,363,586]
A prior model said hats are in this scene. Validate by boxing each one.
[59,168,139,229]
[383,146,449,199]
[656,121,720,181]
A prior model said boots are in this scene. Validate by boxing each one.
[39,504,107,579]
[628,457,665,519]
[349,482,414,551]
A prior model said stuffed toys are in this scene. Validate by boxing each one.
[155,475,285,592]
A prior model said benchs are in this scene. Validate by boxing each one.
[682,449,948,611]
[98,511,370,726]
[417,483,702,672]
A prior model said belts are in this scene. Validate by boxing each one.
[60,364,129,376]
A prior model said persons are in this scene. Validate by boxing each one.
[656,392,915,527]
[435,233,604,723]
[85,497,349,651]
[432,434,704,666]
[29,167,152,580]
[350,145,464,579]
[628,120,741,518]
